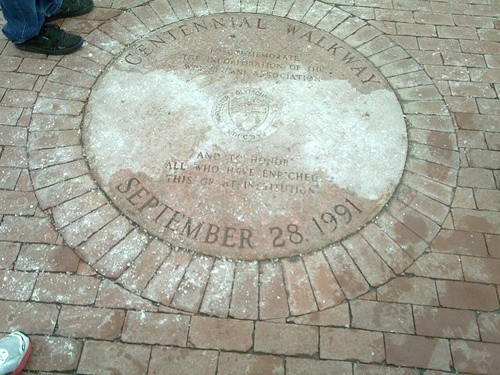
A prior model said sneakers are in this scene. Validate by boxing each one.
[43,0,94,23]
[14,23,83,54]
[0,330,32,375]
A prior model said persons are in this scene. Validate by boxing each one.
[0,0,96,58]
